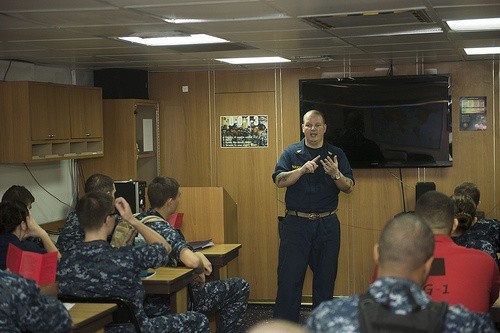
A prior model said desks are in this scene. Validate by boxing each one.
[61,242,242,333]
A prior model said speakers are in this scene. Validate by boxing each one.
[114,179,147,214]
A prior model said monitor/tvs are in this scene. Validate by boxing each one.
[298,72,454,167]
[93,68,149,99]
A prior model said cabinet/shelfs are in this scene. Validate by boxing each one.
[0,82,160,199]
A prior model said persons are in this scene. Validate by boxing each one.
[453,180,500,253]
[306,212,498,333]
[0,200,61,271]
[448,192,500,268]
[0,268,73,333]
[53,191,211,333]
[2,185,49,254]
[368,191,500,316]
[130,176,250,333]
[272,110,355,326]
[64,174,122,227]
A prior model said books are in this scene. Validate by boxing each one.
[188,239,214,250]
[137,271,157,279]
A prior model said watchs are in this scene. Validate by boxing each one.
[333,172,341,180]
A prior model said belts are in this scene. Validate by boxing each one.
[284,209,337,221]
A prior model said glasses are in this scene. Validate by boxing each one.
[104,211,118,223]
